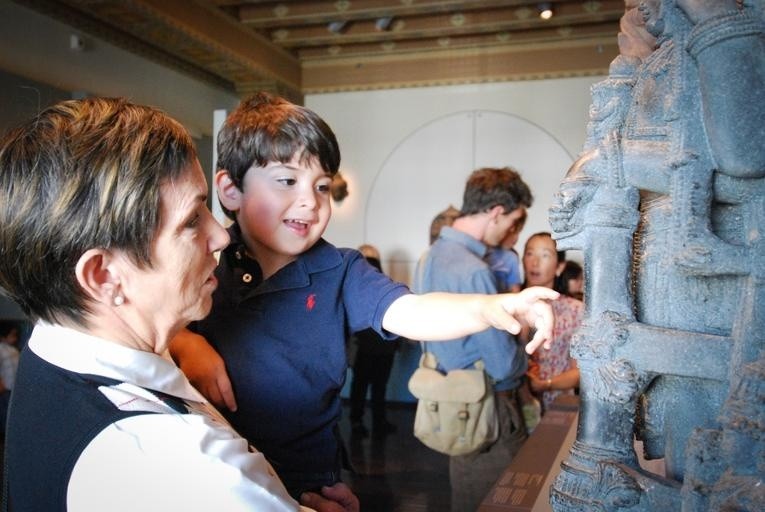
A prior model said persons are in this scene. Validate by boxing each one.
[0,320,21,467]
[0,98,362,511]
[166,90,560,511]
[346,167,587,511]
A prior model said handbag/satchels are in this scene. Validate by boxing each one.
[407,350,500,458]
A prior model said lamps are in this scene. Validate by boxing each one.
[328,20,345,32]
[538,2,553,21]
[374,18,392,29]
